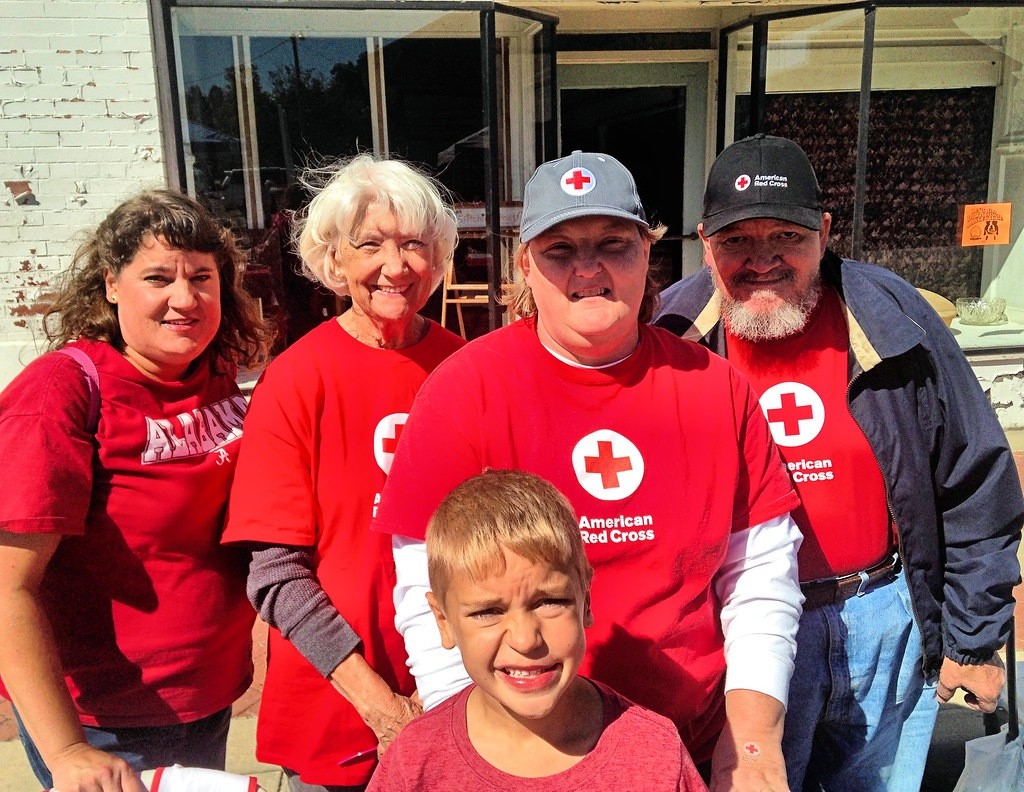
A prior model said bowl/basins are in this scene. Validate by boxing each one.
[956,297,1007,326]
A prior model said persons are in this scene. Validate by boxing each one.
[0,190,279,792]
[374,148,805,792]
[219,151,470,792]
[362,470,710,792]
[648,134,1024,792]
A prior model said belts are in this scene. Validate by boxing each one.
[800,557,900,612]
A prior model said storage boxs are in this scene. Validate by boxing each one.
[452,201,523,227]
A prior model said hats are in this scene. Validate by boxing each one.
[517,148,650,245]
[700,132,825,238]
